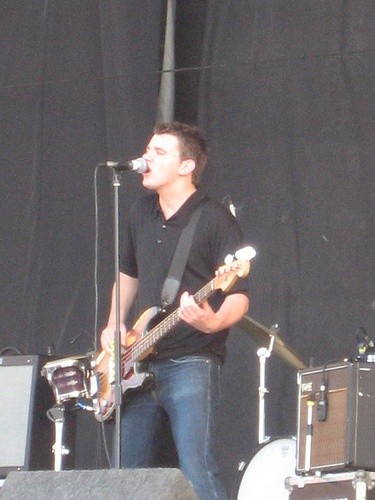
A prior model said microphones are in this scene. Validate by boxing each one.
[318,377,327,421]
[106,158,147,173]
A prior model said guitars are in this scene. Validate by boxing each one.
[83,244,258,421]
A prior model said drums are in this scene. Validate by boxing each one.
[231,429,301,499]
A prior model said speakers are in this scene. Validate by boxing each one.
[0,468,201,500]
[296,360,375,474]
[0,354,77,478]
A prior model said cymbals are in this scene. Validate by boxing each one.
[234,312,307,371]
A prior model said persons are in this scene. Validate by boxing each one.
[101,121,249,500]
